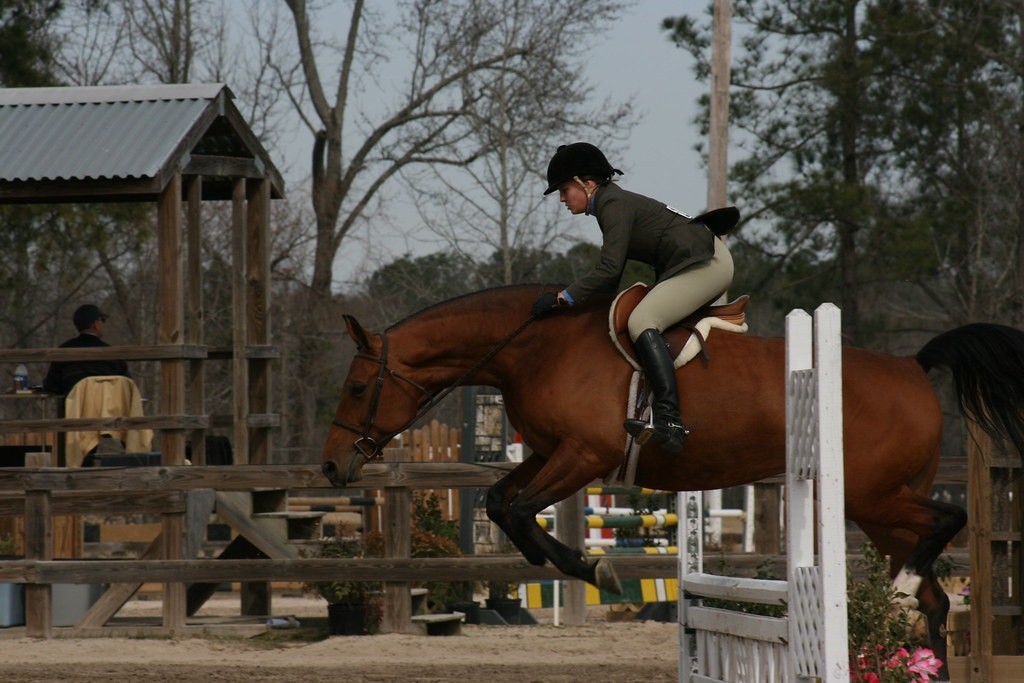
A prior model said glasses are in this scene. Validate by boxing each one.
[95,317,107,325]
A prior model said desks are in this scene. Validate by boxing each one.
[0,394,65,468]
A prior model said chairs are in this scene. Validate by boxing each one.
[64,375,155,467]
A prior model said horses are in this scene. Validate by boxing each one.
[320,280,1022,683]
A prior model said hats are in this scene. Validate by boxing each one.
[73,304,110,326]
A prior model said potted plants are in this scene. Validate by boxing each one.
[295,520,385,634]
[411,490,521,626]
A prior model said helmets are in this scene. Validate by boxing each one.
[543,142,623,196]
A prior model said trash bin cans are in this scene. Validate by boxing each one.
[0,554,27,629]
[51,558,104,627]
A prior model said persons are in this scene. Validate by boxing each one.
[43,303,132,393]
[530,142,741,455]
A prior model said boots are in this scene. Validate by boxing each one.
[624,329,690,454]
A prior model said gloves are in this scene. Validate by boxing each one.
[530,291,564,319]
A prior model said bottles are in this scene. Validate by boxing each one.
[14,363,28,393]
